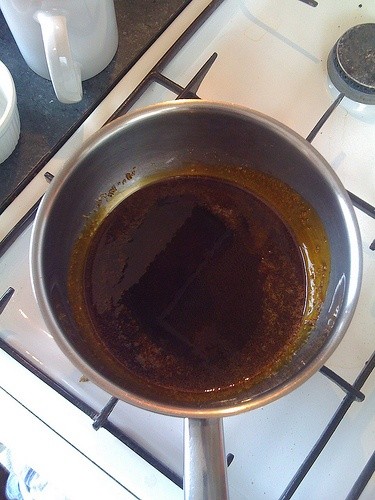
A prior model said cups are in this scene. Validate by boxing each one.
[1,0,121,103]
[1,58,23,164]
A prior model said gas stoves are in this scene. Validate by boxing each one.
[0,7,372,500]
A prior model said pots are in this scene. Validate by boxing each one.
[25,98,364,499]
